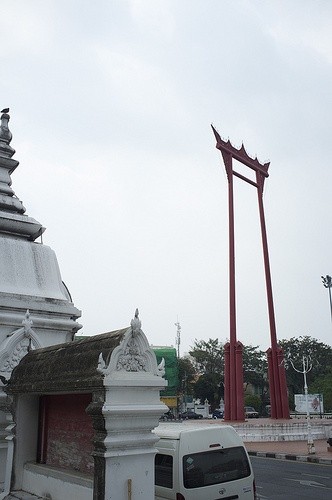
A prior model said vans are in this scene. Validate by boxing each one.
[152,423,257,500]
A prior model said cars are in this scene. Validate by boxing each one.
[158,406,258,421]
